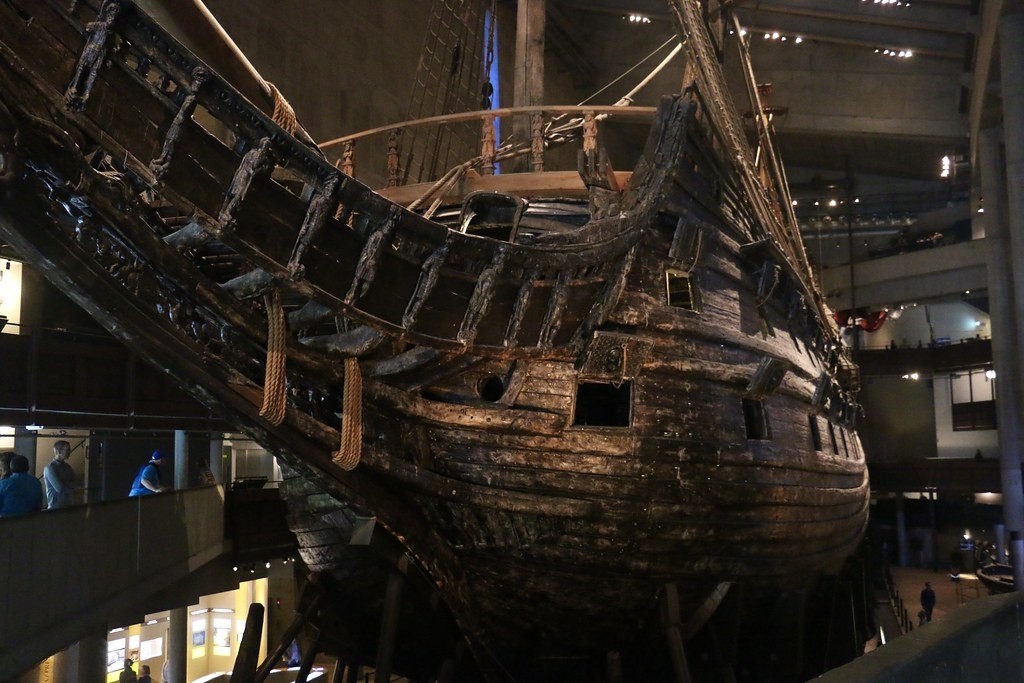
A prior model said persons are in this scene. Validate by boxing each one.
[918,610,926,627]
[531,115,545,161]
[482,118,494,159]
[582,114,597,157]
[138,665,151,683]
[43,440,84,509]
[921,582,936,626]
[128,450,168,497]
[282,636,303,668]
[387,133,400,179]
[0,451,43,519]
[120,659,137,683]
[343,144,354,178]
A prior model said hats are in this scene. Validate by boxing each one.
[151,451,167,460]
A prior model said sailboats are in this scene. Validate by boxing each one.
[1,1,873,683]
